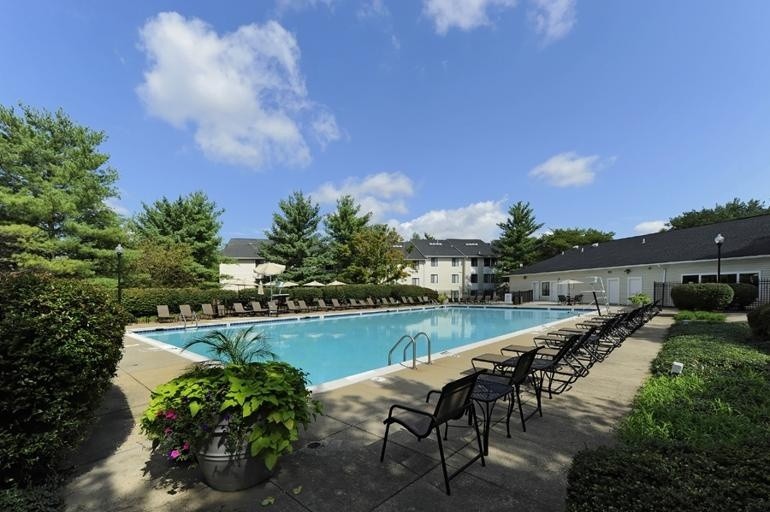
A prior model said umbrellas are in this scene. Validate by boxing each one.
[556,277,584,304]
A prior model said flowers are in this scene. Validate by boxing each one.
[138,325,326,494]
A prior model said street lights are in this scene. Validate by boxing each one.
[115,243,124,306]
[714,233,725,283]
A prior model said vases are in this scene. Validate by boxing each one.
[193,414,278,492]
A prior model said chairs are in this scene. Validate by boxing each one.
[457,289,584,307]
[150,293,435,323]
[374,297,665,498]
